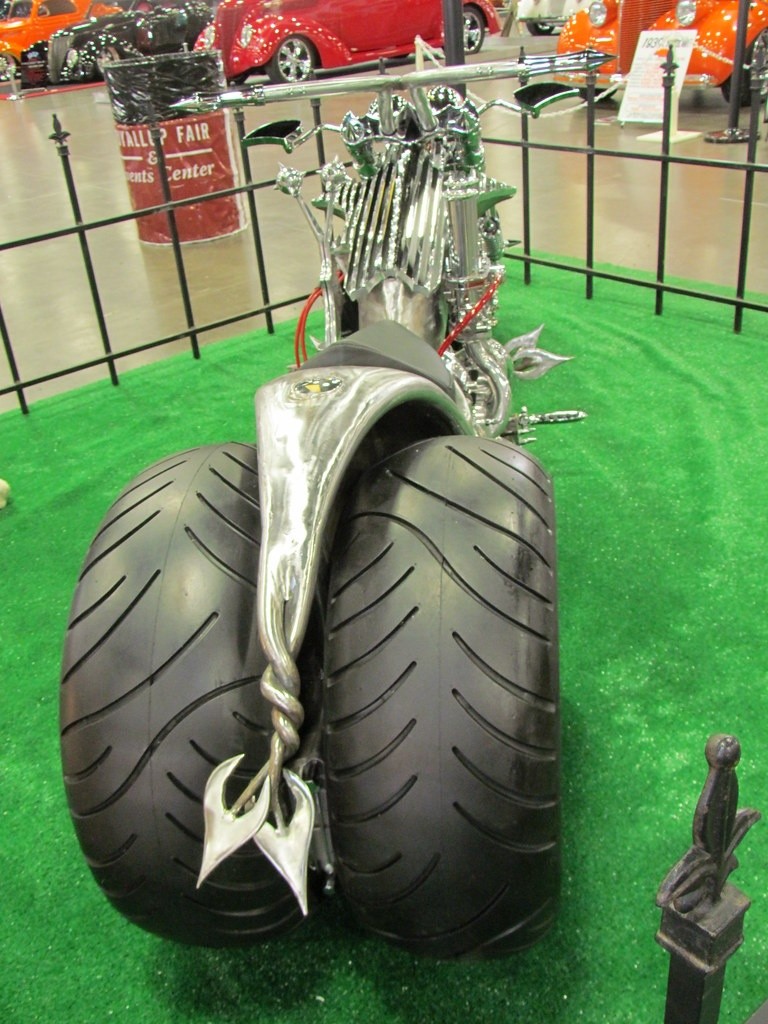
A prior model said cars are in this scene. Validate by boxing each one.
[48,0,214,83]
[0,0,124,82]
[193,0,503,83]
[552,0,768,107]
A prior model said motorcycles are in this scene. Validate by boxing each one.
[58,48,618,957]
